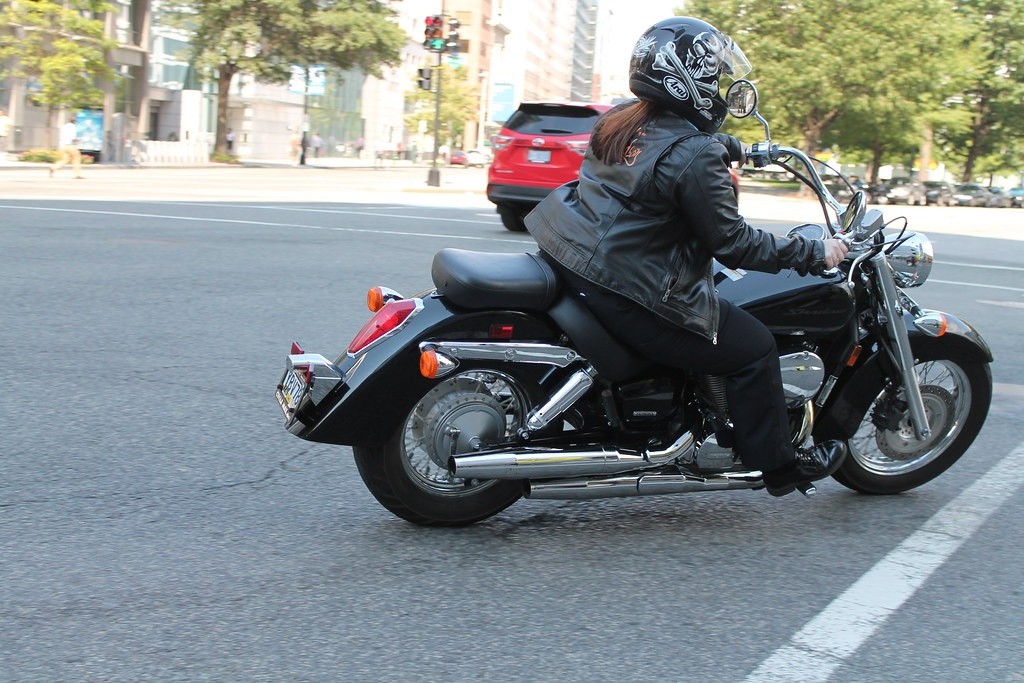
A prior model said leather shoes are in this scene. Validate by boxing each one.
[763,439,849,497]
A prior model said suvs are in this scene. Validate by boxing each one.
[487,99,621,235]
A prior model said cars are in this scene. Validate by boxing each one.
[838,178,1024,209]
[449,151,466,165]
[468,149,484,167]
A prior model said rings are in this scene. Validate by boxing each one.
[834,258,839,263]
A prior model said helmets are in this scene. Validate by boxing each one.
[630,15,752,134]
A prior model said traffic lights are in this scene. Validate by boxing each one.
[422,14,444,53]
[417,68,432,90]
[447,17,461,52]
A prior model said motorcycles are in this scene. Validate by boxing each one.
[273,77,997,531]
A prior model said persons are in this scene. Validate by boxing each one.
[48,117,86,180]
[226,130,449,164]
[523,17,848,497]
